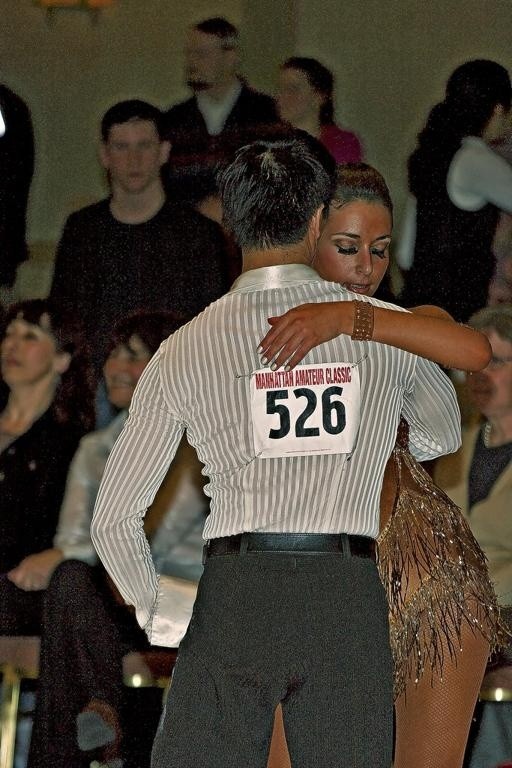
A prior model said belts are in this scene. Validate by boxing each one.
[203,531,379,565]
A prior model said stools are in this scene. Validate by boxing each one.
[0,635,41,765]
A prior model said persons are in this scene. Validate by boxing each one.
[1,15,512,768]
